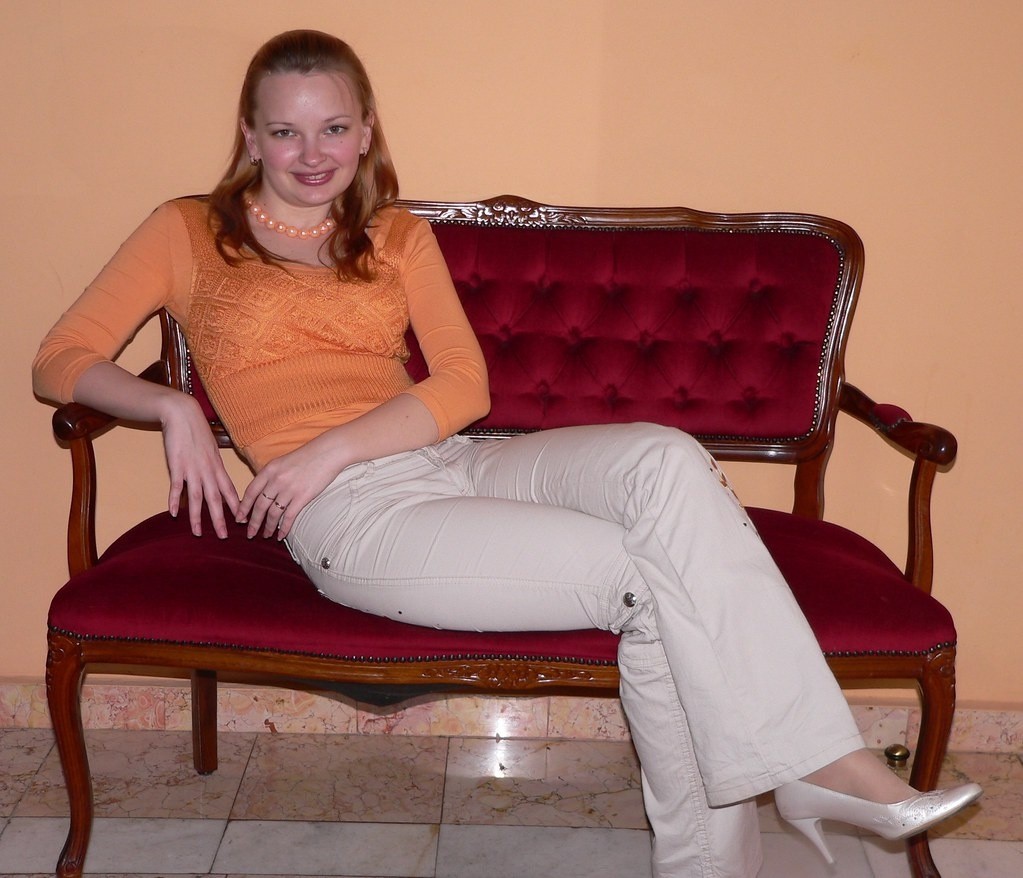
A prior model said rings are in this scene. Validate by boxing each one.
[273,500,287,511]
[262,492,276,500]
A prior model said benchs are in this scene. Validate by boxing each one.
[41,195,957,878]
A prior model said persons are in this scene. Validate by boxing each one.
[30,30,981,878]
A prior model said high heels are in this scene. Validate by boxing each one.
[774,779,985,865]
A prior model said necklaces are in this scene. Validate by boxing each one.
[244,193,335,237]
